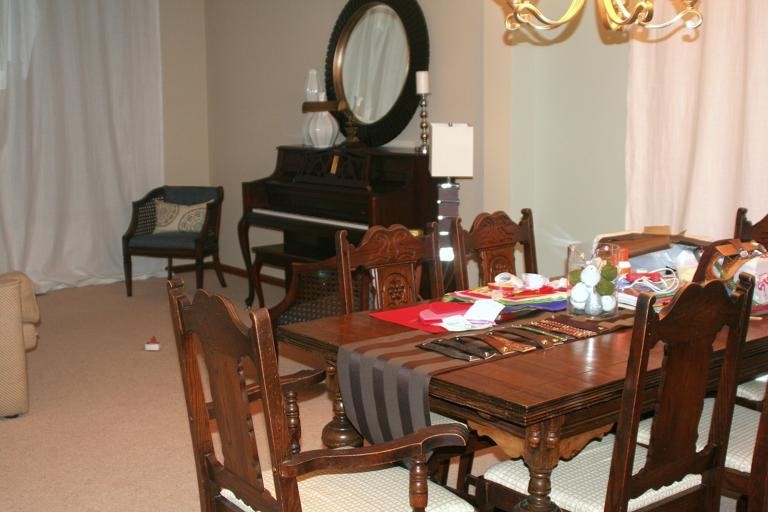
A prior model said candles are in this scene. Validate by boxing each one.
[412,68,429,92]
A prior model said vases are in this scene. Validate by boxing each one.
[304,91,337,152]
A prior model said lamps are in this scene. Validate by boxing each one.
[426,121,475,262]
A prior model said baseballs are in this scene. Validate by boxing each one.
[570,262,618,316]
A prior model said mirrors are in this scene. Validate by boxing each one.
[327,2,431,150]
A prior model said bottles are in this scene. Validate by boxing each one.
[618,249,632,274]
[309,93,340,149]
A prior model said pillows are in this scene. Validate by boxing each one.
[151,198,214,234]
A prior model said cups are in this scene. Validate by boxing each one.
[566,244,621,320]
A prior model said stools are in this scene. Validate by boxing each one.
[0,271,41,418]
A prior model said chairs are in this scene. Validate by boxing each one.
[122,184,227,297]
[452,208,537,290]
[634,386,768,511]
[335,223,496,496]
[732,208,767,411]
[166,277,479,512]
[481,272,754,512]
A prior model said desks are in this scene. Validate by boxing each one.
[276,272,768,512]
